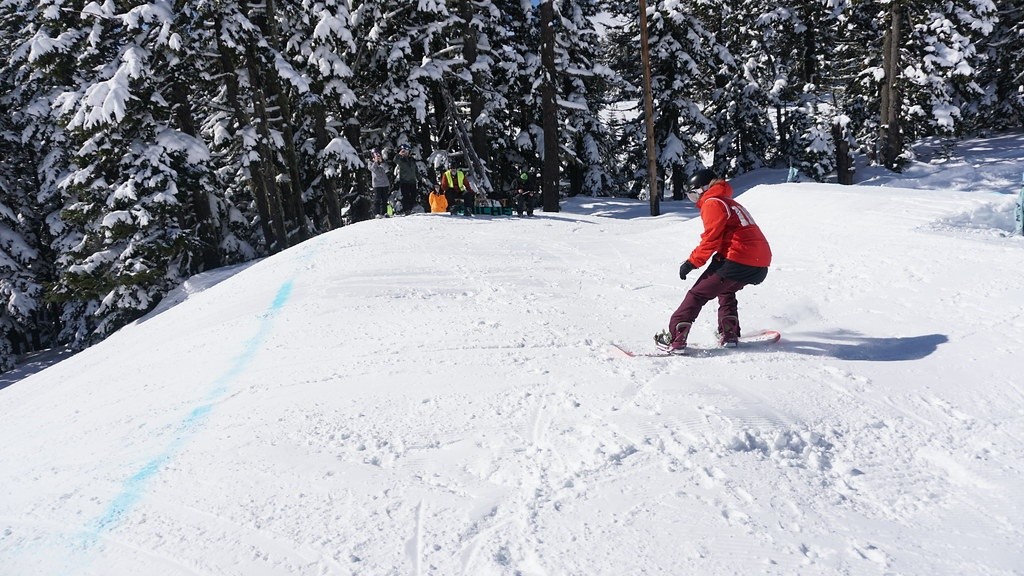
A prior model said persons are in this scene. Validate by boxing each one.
[510,173,540,217]
[367,144,476,219]
[654,169,771,354]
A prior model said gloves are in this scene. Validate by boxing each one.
[680,259,697,280]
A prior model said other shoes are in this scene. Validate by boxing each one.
[516,213,523,218]
[527,214,537,218]
[375,213,382,219]
[384,213,393,218]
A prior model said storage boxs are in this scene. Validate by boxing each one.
[455,199,511,216]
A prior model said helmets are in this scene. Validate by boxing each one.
[680,169,718,194]
[398,144,411,150]
[371,148,382,157]
[448,161,458,169]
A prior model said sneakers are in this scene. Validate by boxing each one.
[716,315,741,347]
[653,322,692,355]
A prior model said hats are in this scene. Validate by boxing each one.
[520,173,528,180]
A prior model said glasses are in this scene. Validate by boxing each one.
[450,167,455,170]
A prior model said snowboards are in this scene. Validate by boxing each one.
[613,330,780,358]
